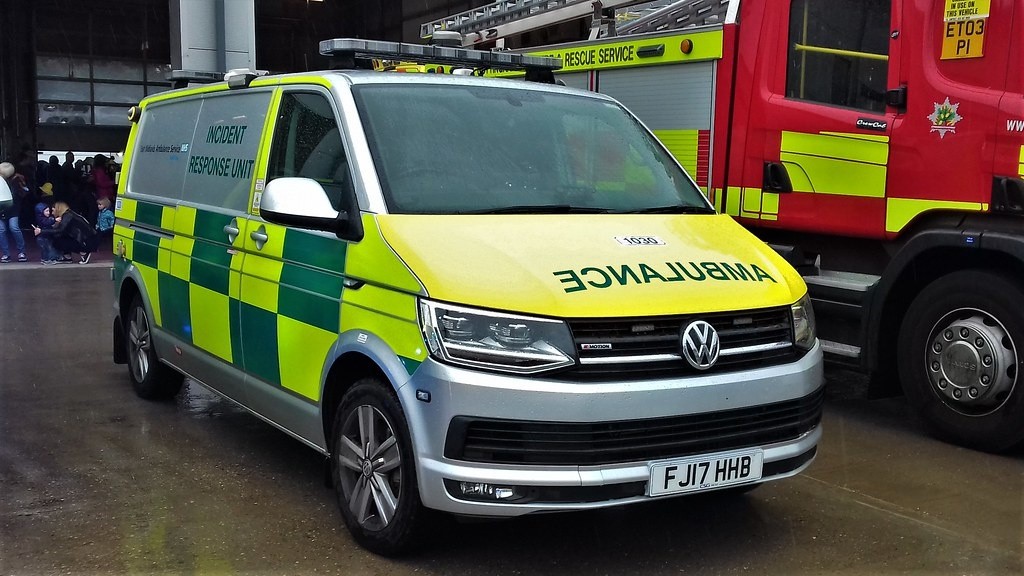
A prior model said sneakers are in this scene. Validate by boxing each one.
[56,256,74,264]
[78,252,91,264]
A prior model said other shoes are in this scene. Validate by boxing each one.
[41,258,48,265]
[0,254,12,263]
[18,253,28,262]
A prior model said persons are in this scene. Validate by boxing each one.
[0,152,119,264]
[94,196,115,253]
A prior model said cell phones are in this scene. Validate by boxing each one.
[31,224,36,229]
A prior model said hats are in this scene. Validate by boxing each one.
[39,182,54,196]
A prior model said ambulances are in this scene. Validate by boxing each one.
[106,36,827,563]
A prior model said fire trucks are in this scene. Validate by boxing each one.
[406,1,1024,459]
